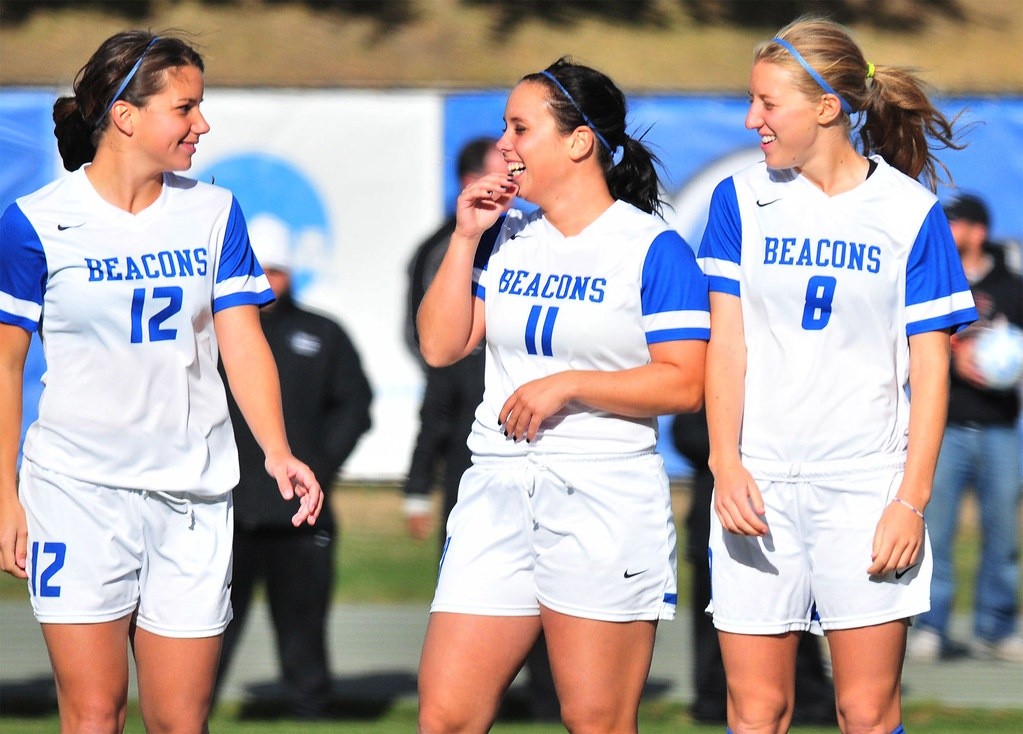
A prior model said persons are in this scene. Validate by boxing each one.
[412,60,711,734]
[215,214,370,716]
[915,193,1023,665]
[401,137,560,706]
[695,18,976,734]
[0,31,323,734]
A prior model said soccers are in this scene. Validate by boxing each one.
[971,320,1023,390]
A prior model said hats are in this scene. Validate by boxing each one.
[943,197,987,226]
[246,213,293,274]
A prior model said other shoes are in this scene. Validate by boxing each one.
[911,629,942,664]
[972,632,1023,663]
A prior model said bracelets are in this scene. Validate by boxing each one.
[894,498,924,519]
